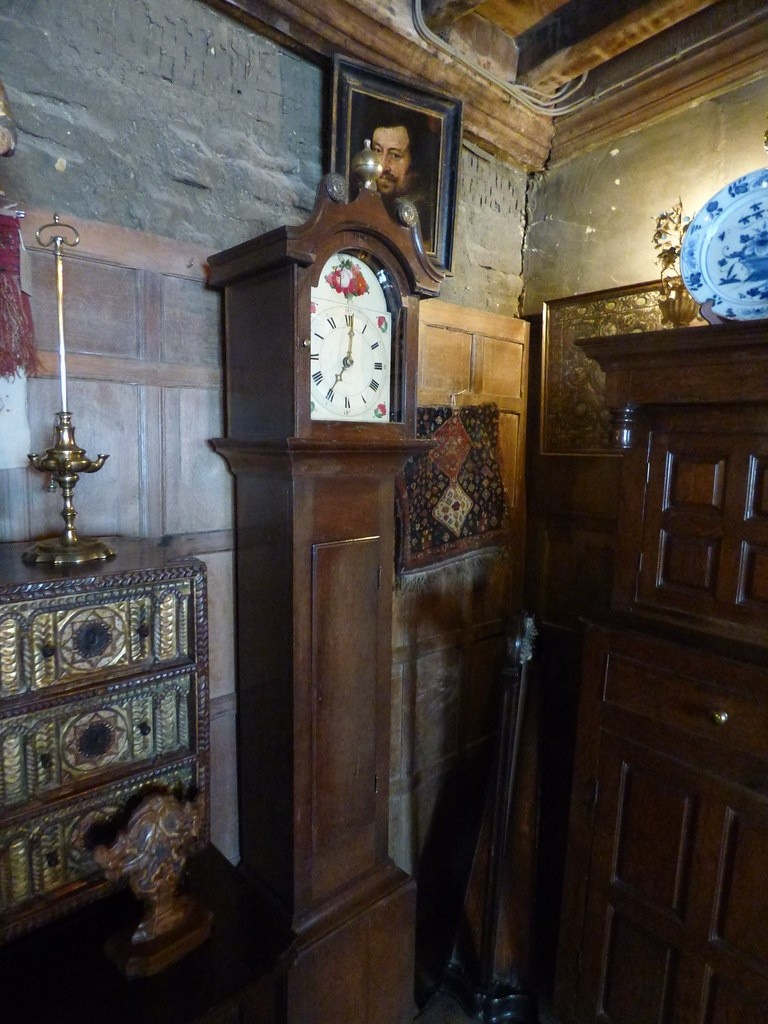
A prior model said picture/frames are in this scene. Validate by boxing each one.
[321,49,463,274]
[536,275,711,460]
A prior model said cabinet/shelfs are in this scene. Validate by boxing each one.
[534,318,768,1024]
[0,535,210,946]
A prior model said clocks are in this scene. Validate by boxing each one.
[311,251,392,423]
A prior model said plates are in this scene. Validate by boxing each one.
[679,168,768,321]
